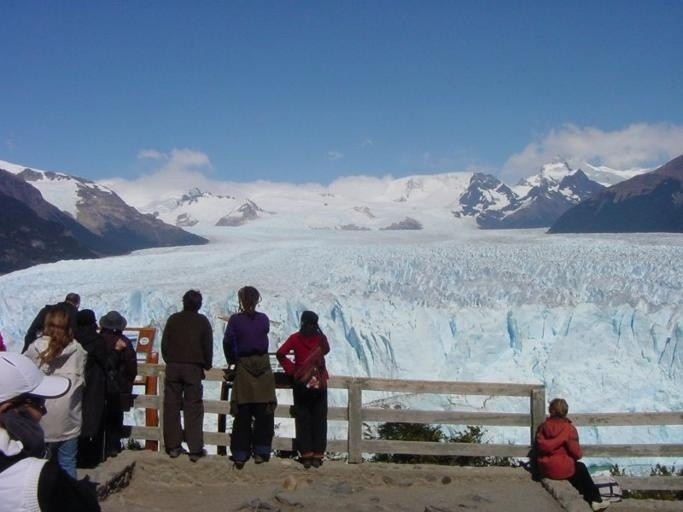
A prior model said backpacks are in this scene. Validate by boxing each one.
[589,469,622,502]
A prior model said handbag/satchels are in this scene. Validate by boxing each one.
[293,359,323,393]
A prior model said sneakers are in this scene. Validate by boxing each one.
[169,445,187,457]
[591,499,611,511]
[188,449,207,462]
[236,460,244,467]
[296,455,324,468]
[254,453,265,463]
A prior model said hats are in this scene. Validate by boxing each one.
[80,309,98,330]
[99,310,127,330]
[0,351,71,405]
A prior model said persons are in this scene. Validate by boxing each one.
[535,399,610,511]
[161,290,213,462]
[277,311,330,469]
[223,286,277,470]
[0,293,138,512]
